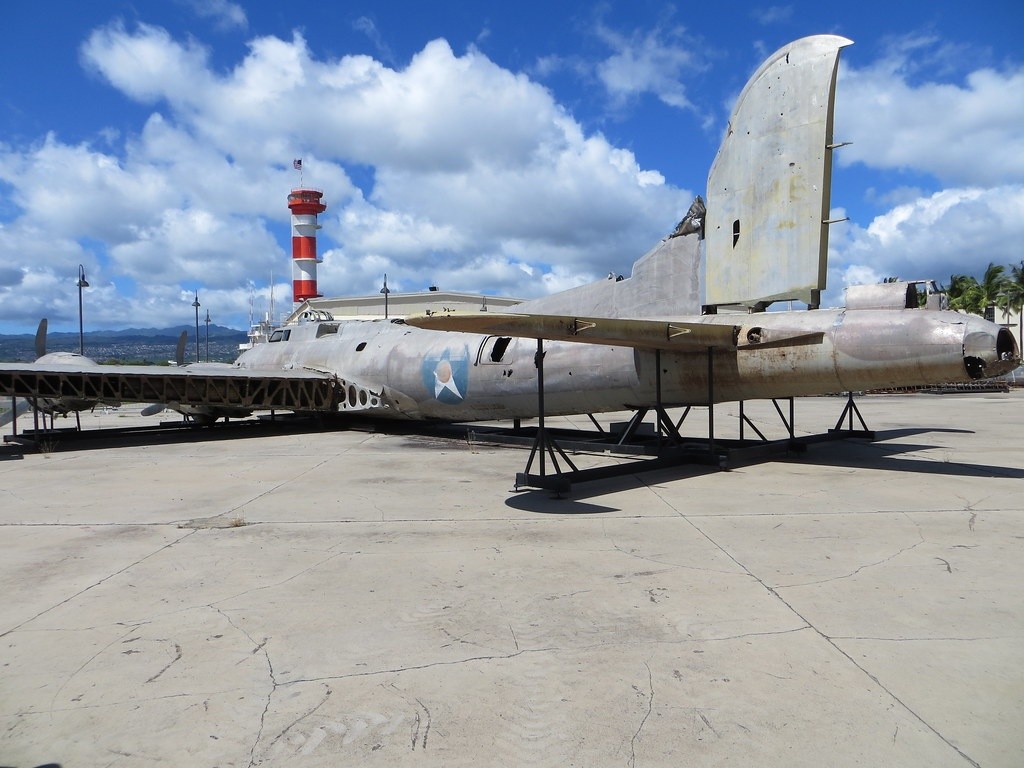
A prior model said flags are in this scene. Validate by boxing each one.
[293,158,302,170]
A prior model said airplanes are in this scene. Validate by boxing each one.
[1,33,1018,495]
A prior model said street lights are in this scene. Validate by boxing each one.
[193,289,200,362]
[380,273,390,320]
[76,263,89,354]
[204,309,211,363]
